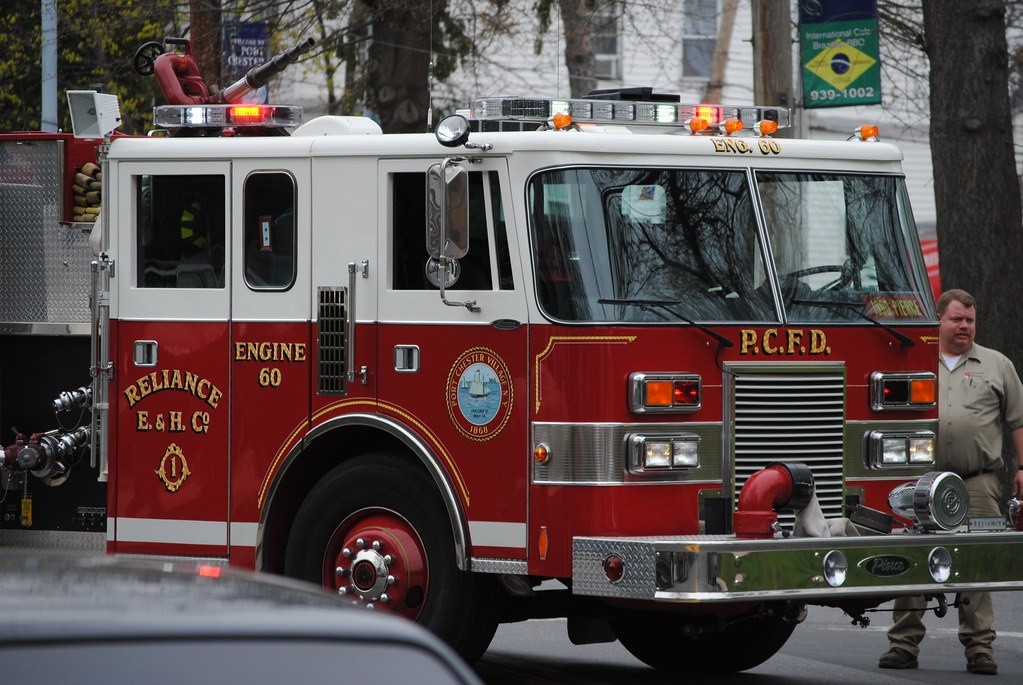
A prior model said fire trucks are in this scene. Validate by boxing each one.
[66,34,1019,674]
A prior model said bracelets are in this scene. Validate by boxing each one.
[1018,465,1023,470]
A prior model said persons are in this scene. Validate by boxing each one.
[878,288,1023,675]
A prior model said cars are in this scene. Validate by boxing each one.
[0,548,484,685]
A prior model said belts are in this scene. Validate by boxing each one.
[959,468,994,480]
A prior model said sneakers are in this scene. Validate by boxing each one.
[967,653,998,674]
[879,647,918,669]
